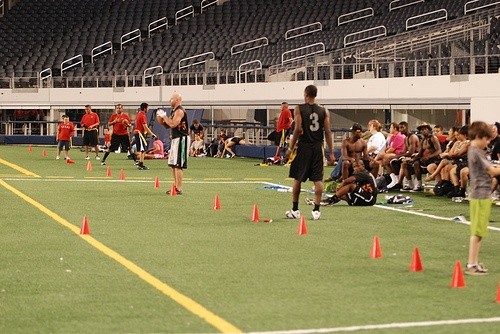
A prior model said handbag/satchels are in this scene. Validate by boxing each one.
[433,179,454,196]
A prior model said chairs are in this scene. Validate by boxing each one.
[0,0,500,83]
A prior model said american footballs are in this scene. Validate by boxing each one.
[157,114,165,125]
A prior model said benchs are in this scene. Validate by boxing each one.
[56,136,105,146]
[226,144,264,159]
[264,145,341,161]
[0,134,55,145]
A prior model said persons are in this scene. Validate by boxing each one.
[305,120,500,206]
[285,85,335,219]
[101,104,138,165]
[466,121,500,275]
[267,102,297,164]
[128,134,164,159]
[80,105,100,159]
[189,120,245,158]
[56,115,74,159]
[156,94,189,194]
[134,102,157,170]
[91,128,110,152]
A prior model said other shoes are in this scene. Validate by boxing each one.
[65,157,70,160]
[101,161,106,165]
[134,161,139,165]
[231,154,235,158]
[167,188,183,194]
[320,198,330,207]
[448,190,465,198]
[465,265,489,275]
[95,155,101,160]
[85,156,90,159]
[56,156,60,159]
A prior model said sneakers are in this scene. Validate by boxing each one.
[137,165,150,169]
[285,209,300,219]
[312,209,320,219]
[409,185,424,192]
[400,185,413,192]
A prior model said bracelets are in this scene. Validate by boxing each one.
[288,148,292,151]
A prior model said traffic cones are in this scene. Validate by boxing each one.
[249,203,260,222]
[154,176,160,188]
[212,195,221,210]
[42,147,47,156]
[86,160,93,171]
[369,235,383,259]
[79,215,91,235]
[170,185,176,196]
[106,166,111,176]
[494,282,500,303]
[66,158,74,164]
[449,259,465,288]
[119,169,126,180]
[297,214,308,235]
[28,145,32,152]
[409,246,423,272]
